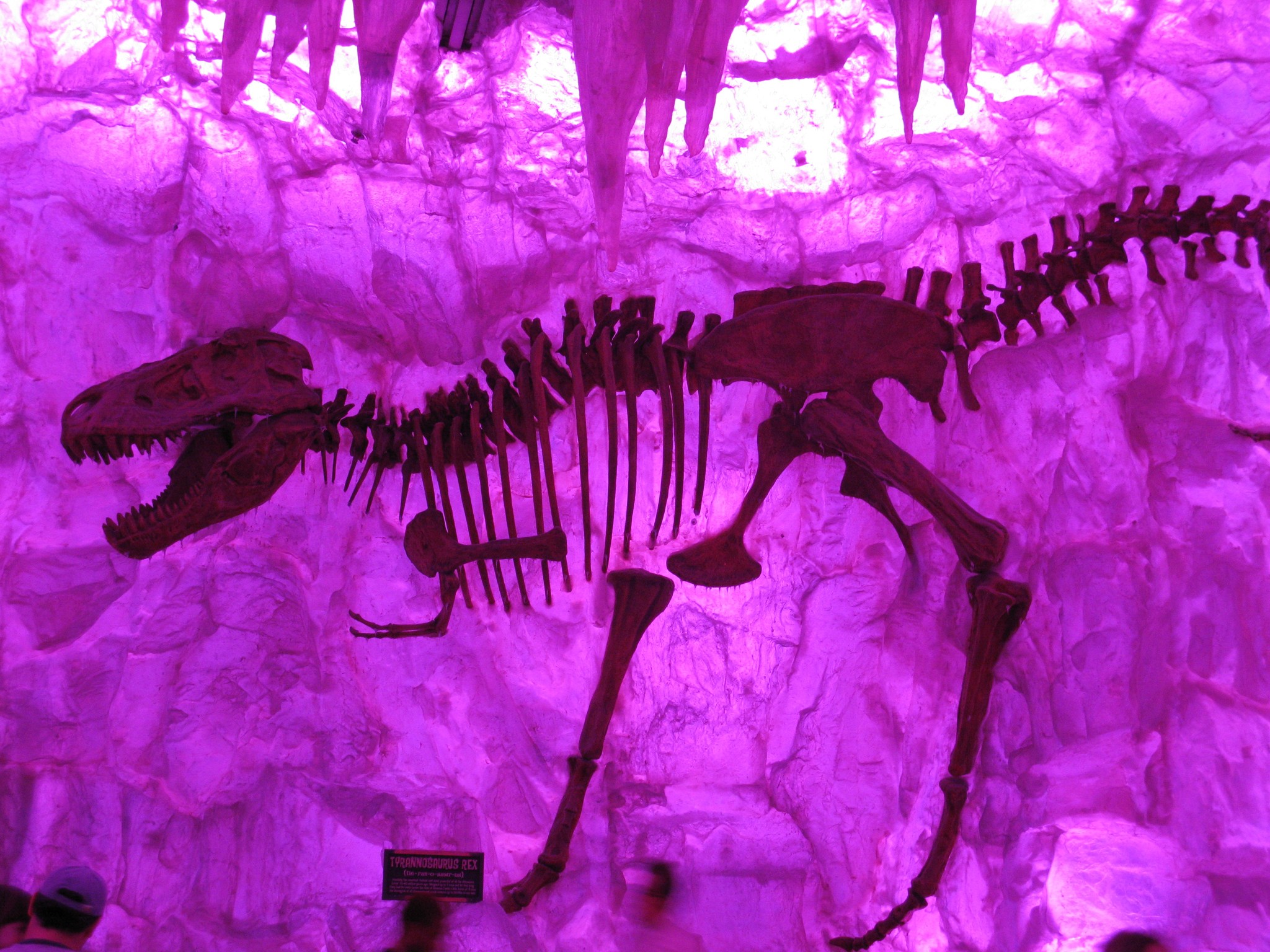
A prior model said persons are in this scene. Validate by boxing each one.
[0,884,33,948]
[1104,931,1168,952]
[0,865,107,952]
[381,895,453,952]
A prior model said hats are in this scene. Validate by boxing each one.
[39,866,107,917]
